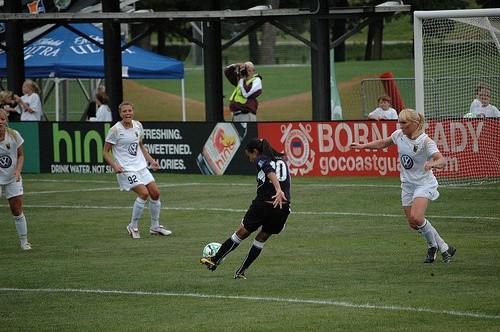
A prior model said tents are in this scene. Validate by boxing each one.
[0,23,187,122]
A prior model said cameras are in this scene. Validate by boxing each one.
[239,65,247,80]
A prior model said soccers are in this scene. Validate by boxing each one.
[203,242,225,265]
[463,112,478,118]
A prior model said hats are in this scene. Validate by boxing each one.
[479,89,491,99]
[245,62,255,71]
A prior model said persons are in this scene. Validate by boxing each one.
[224,61,256,120]
[369,95,401,119]
[89,93,114,121]
[103,101,174,238]
[5,78,41,121]
[0,90,20,122]
[465,82,500,118]
[0,109,32,250]
[200,138,290,280]
[80,85,106,121]
[350,108,457,263]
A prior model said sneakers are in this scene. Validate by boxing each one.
[150,225,172,236]
[20,244,31,250]
[126,223,141,238]
[423,243,439,263]
[200,258,217,272]
[442,247,456,263]
[233,274,249,280]
